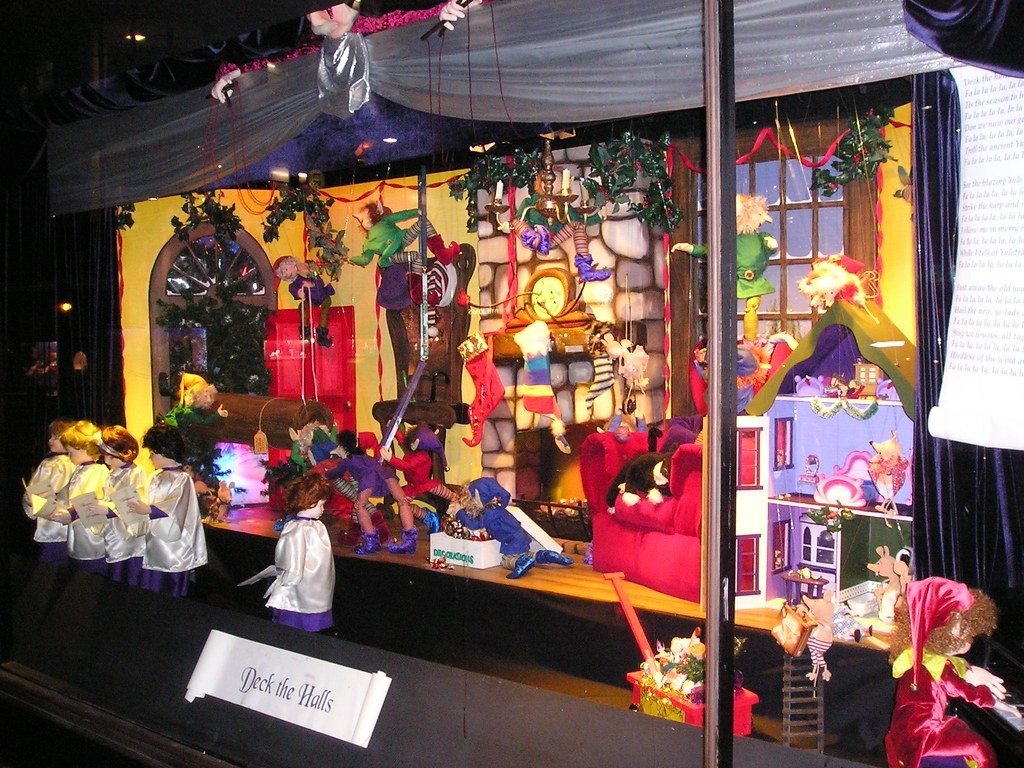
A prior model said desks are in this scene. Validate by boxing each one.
[798,475,818,499]
[781,573,830,604]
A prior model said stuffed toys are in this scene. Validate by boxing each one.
[607,453,671,513]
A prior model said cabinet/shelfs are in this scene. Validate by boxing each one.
[760,398,912,606]
[853,364,884,395]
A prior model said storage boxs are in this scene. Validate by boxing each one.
[429,532,504,569]
[626,671,759,736]
[0,551,870,768]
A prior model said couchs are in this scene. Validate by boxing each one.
[578,430,703,601]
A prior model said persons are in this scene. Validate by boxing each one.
[272,256,335,348]
[446,478,574,579]
[0,334,87,559]
[161,371,229,458]
[671,194,778,348]
[22,420,210,627]
[263,472,337,633]
[512,170,616,284]
[289,417,458,555]
[343,203,461,305]
[884,576,1024,768]
